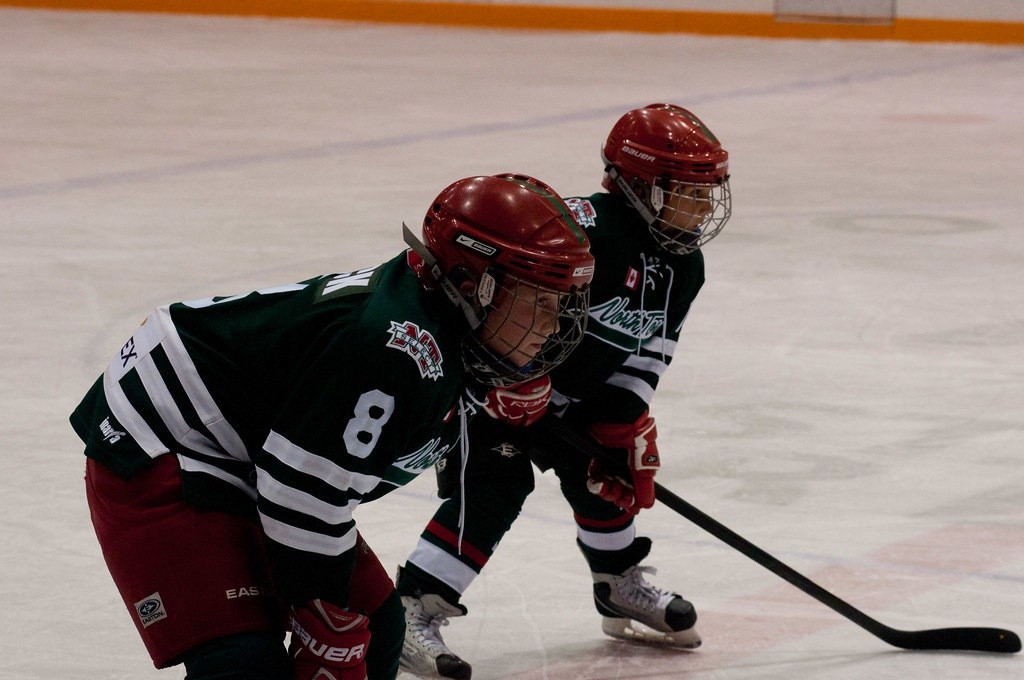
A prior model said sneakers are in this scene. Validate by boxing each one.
[576,536,702,650]
[394,564,472,680]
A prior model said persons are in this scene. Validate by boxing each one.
[387,104,732,680]
[69,173,596,680]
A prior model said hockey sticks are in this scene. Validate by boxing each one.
[613,463,1024,655]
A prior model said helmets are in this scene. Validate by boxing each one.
[603,101,731,256]
[422,173,595,391]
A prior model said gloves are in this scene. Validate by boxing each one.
[287,598,370,680]
[484,368,553,428]
[586,411,659,516]
